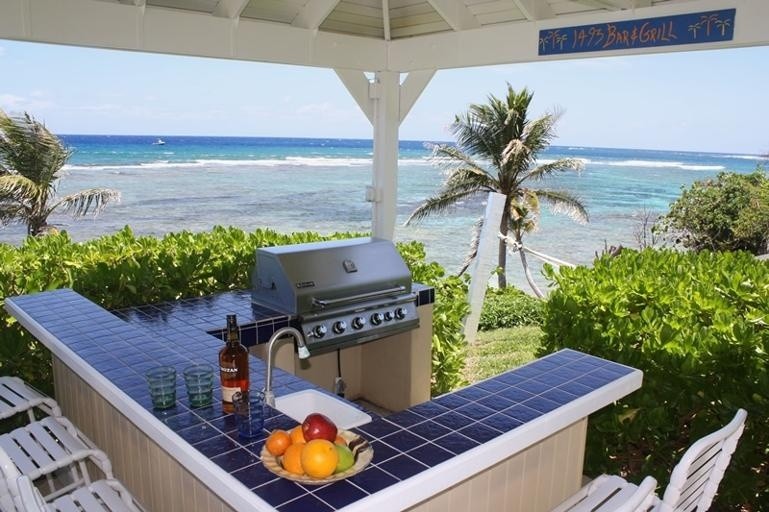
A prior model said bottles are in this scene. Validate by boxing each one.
[218,315,249,415]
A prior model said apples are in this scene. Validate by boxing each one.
[302,413,338,443]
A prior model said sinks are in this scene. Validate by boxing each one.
[276,390,372,430]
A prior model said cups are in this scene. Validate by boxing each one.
[145,364,265,440]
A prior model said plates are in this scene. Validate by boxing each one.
[260,427,373,485]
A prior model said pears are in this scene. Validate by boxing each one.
[335,444,354,473]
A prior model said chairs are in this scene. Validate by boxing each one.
[551,408,748,512]
[614,475,657,512]
[0,375,151,512]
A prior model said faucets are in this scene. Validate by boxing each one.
[266,327,310,390]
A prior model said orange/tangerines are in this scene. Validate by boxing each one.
[266,424,305,476]
